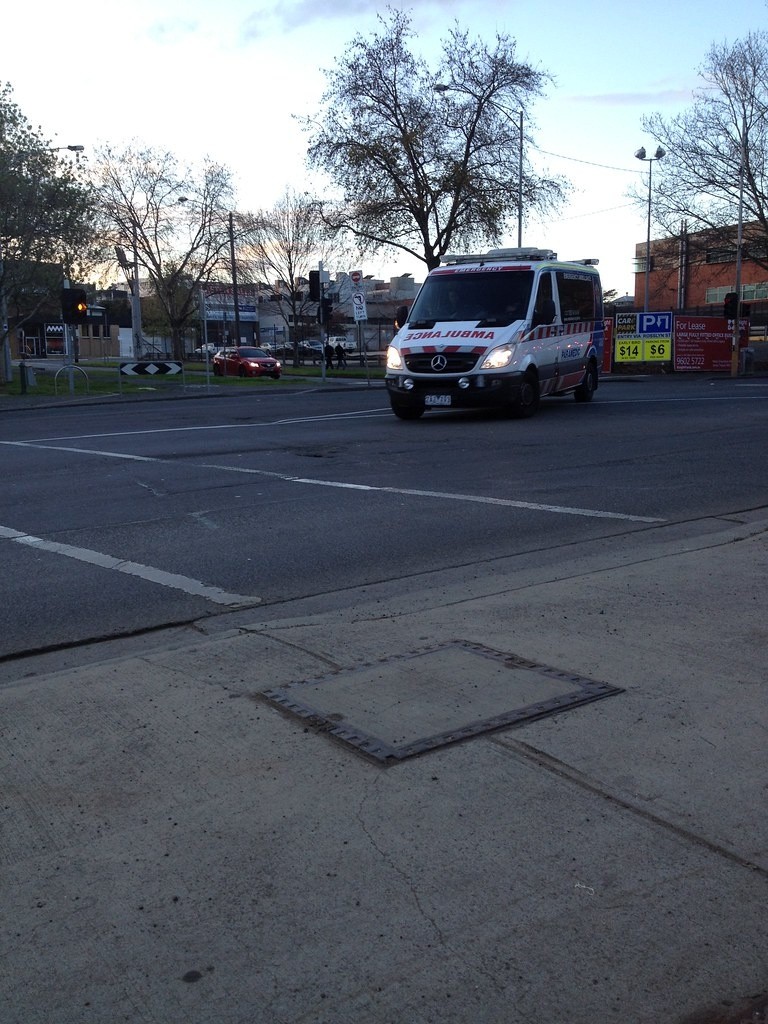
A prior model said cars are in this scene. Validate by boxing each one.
[195,343,236,355]
[212,346,282,379]
[259,340,324,358]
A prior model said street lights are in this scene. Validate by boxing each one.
[430,84,524,247]
[633,145,664,366]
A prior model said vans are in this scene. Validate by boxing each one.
[385,247,606,427]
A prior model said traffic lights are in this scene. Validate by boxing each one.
[740,301,751,318]
[176,197,241,345]
[723,293,738,320]
[309,270,319,301]
[61,287,89,325]
[323,298,333,321]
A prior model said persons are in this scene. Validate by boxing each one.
[435,288,465,321]
[474,291,489,312]
[486,285,522,316]
[335,343,346,370]
[321,342,335,371]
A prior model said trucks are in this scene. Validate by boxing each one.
[324,337,357,354]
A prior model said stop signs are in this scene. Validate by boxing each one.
[352,272,361,282]
